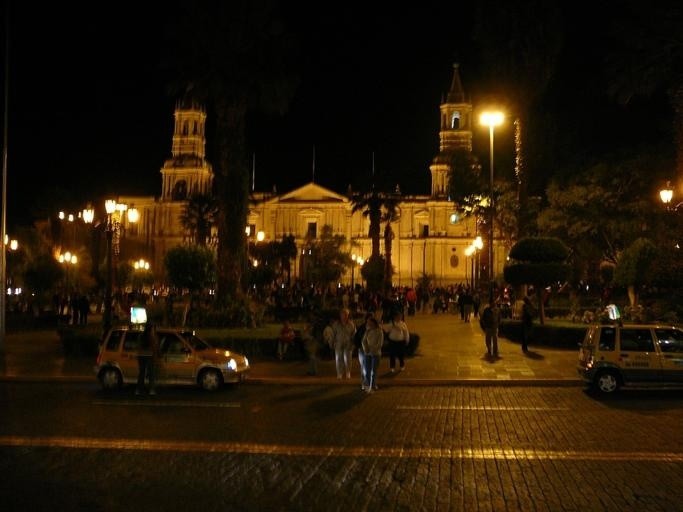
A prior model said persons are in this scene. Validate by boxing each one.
[521,296,532,353]
[479,307,499,361]
[324,308,409,393]
[278,319,295,360]
[247,284,516,319]
[136,324,159,395]
[11,284,218,340]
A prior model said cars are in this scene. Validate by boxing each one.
[580,323,683,394]
[94,328,250,394]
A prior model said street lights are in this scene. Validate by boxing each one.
[479,109,506,305]
[57,211,81,249]
[81,200,126,327]
[135,259,150,291]
[659,186,673,213]
[352,254,363,290]
[59,250,78,313]
[466,237,483,295]
[4,236,17,280]
